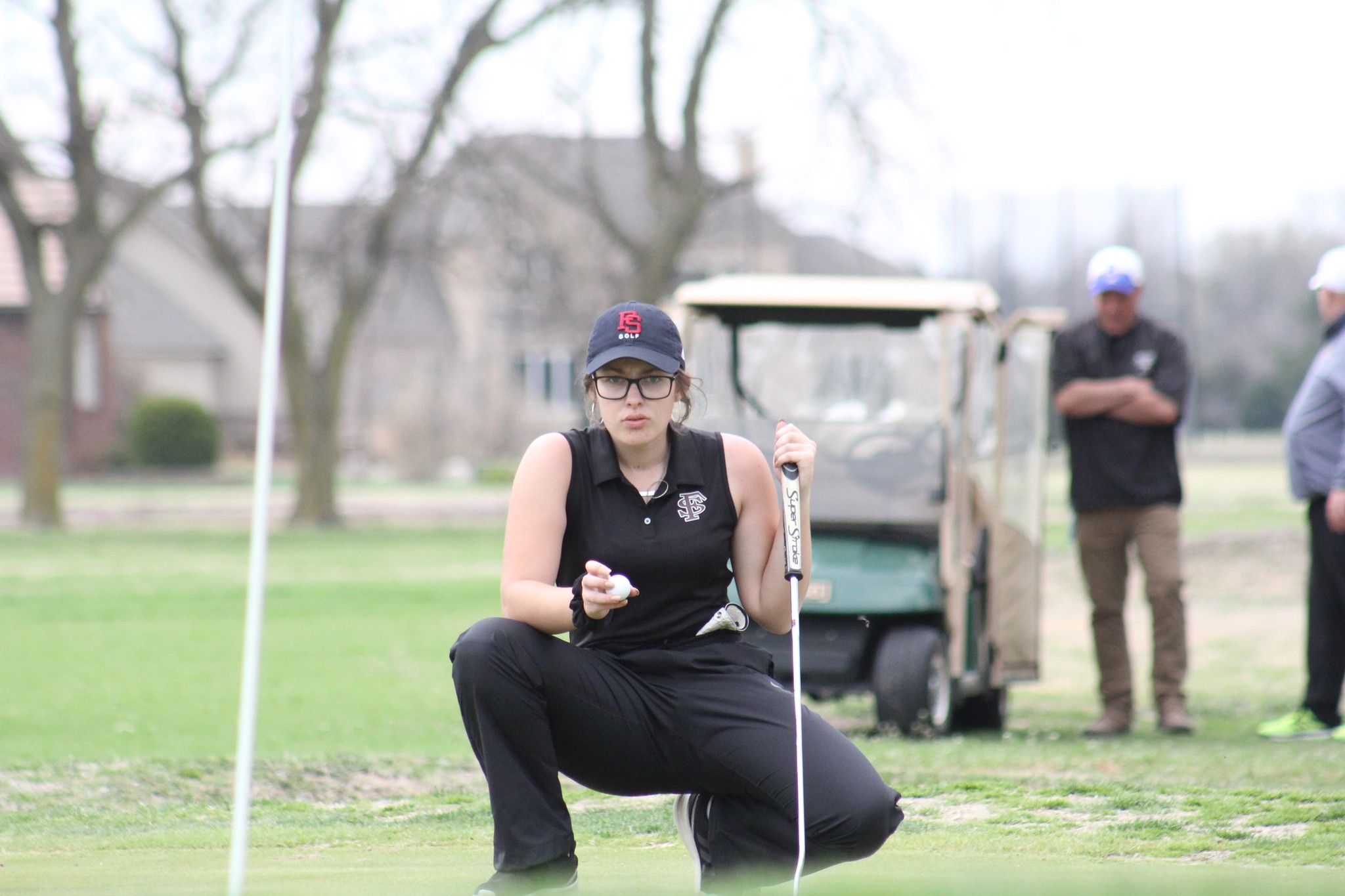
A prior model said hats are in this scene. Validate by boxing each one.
[1087,245,1146,296]
[1308,247,1345,294]
[586,301,686,375]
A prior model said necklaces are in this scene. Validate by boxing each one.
[638,443,668,499]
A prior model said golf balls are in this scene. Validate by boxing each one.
[605,574,632,602]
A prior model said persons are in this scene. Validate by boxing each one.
[1052,247,1193,741]
[449,301,904,896]
[1261,247,1345,740]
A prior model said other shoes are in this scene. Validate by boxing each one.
[1156,696,1192,735]
[1084,708,1130,735]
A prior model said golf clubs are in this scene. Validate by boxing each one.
[779,464,807,895]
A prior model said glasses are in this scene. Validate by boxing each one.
[591,373,679,399]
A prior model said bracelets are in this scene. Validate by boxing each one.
[570,573,603,630]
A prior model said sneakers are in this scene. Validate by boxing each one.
[1257,707,1334,740]
[472,851,578,896]
[674,794,761,896]
